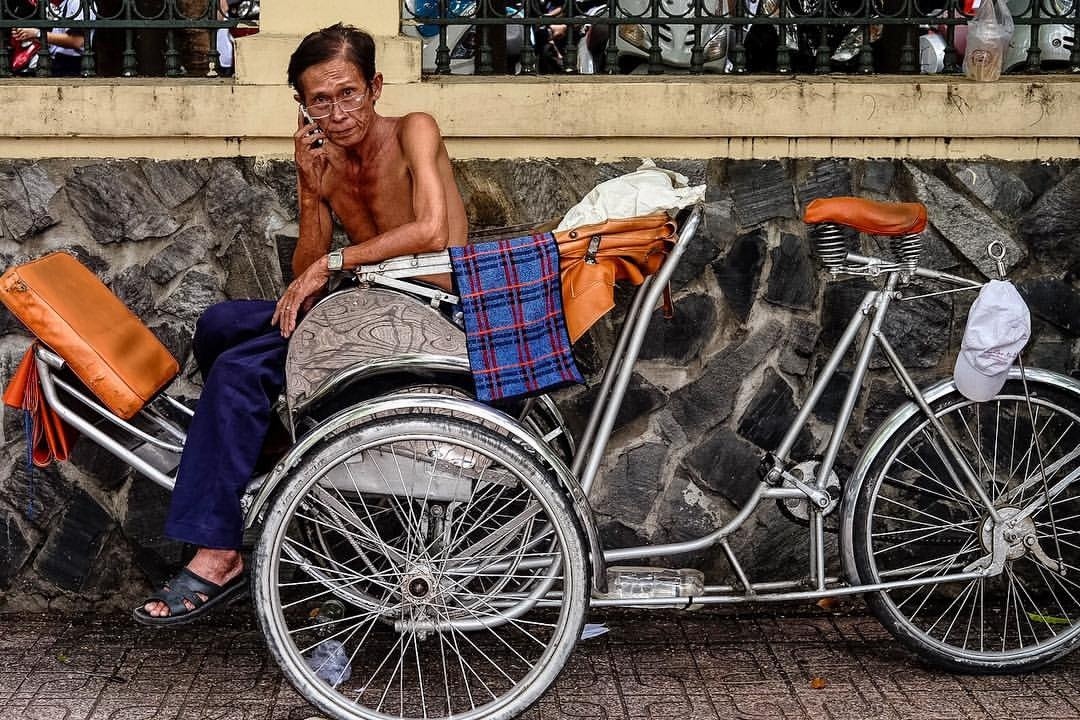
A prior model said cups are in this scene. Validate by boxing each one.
[967,19,1006,83]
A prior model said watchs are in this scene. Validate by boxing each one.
[36,31,41,38]
[327,248,344,272]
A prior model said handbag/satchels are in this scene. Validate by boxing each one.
[962,0,1014,82]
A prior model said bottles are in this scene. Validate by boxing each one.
[591,566,705,611]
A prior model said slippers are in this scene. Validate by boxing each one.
[133,566,247,629]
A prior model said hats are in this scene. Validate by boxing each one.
[954,279,1031,401]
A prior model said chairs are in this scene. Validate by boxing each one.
[283,289,476,407]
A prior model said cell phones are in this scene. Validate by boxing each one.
[298,104,325,147]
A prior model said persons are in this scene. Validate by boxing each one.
[743,0,816,75]
[129,21,467,627]
[216,0,233,77]
[11,0,96,78]
[531,0,594,75]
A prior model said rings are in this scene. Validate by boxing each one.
[286,304,290,309]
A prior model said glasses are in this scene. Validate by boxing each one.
[302,84,369,120]
